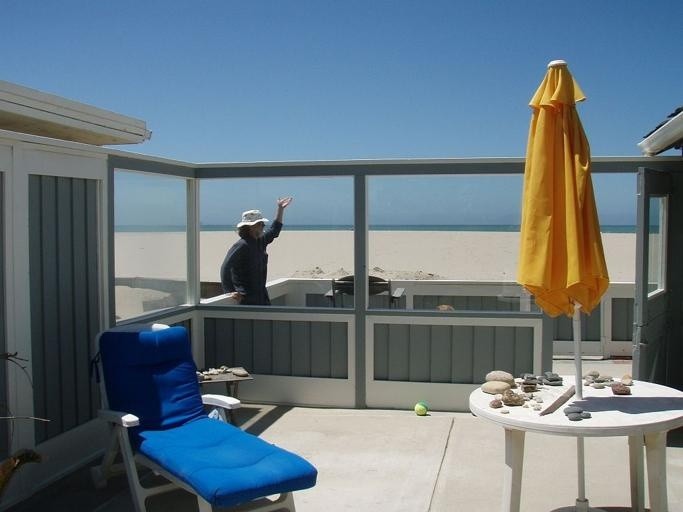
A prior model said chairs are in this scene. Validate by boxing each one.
[93,321,317,511]
[322,274,405,310]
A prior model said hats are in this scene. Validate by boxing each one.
[237,210,269,227]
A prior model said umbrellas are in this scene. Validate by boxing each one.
[513,56,612,512]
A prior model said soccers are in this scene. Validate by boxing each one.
[415,402,428,415]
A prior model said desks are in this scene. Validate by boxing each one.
[191,365,253,405]
[467,373,683,511]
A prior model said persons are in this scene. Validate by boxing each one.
[218,194,295,306]
